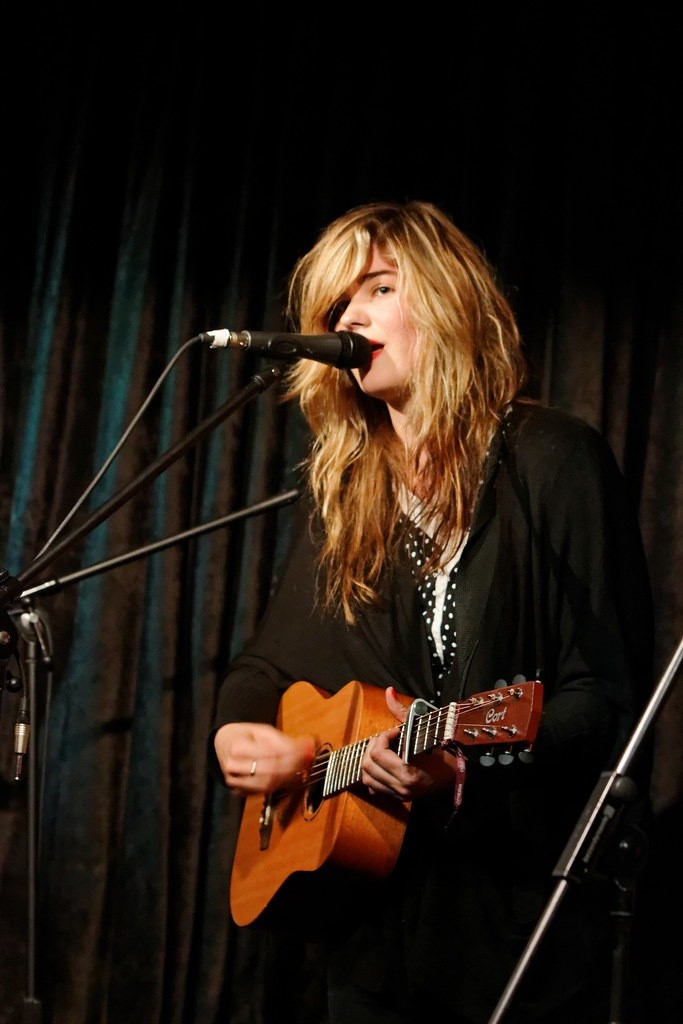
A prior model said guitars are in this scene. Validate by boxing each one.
[229,674,542,929]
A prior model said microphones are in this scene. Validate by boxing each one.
[200,328,372,370]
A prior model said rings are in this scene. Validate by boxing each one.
[250,761,256,776]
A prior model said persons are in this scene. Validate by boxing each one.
[213,201,656,1024]
